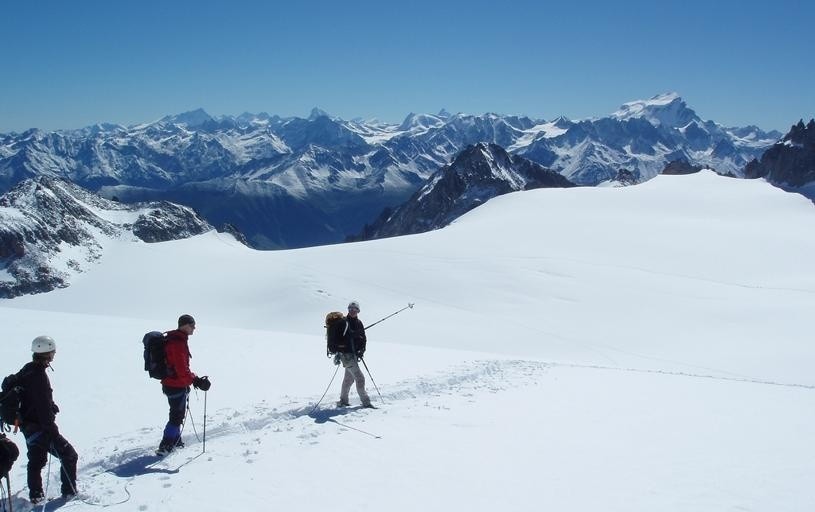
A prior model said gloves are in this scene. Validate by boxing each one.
[192,374,212,392]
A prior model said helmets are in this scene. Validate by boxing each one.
[30,335,57,354]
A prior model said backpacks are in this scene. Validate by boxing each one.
[0,371,28,435]
[141,330,167,380]
[324,310,347,358]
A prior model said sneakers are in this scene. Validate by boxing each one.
[155,442,176,457]
[30,496,54,506]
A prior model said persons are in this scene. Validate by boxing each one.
[0,429,19,482]
[13,335,78,504]
[154,314,210,457]
[333,301,376,409]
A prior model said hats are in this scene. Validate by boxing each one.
[177,314,196,326]
[347,301,360,313]
